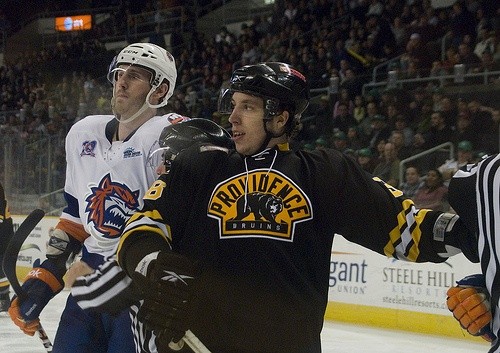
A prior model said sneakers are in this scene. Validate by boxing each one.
[0,289,10,316]
[8,260,64,337]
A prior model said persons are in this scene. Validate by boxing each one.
[0,39,122,193]
[165,0,294,120]
[0,184,14,316]
[447,152,500,353]
[62,0,226,39]
[9,42,192,353]
[117,63,480,353]
[291,0,500,211]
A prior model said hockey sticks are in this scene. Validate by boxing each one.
[1,208,53,353]
[181,328,212,353]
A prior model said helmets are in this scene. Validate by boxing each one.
[159,118,235,172]
[107,41,176,95]
[230,62,311,121]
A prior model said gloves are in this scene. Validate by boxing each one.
[138,253,201,352]
[447,274,493,337]
[433,213,477,262]
